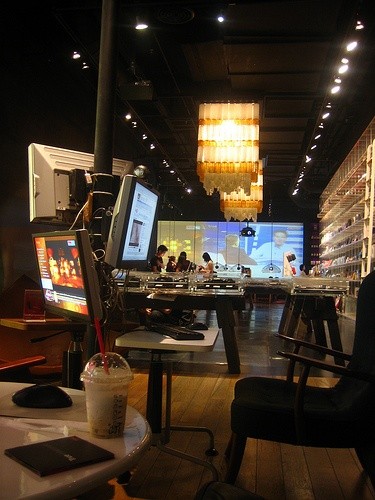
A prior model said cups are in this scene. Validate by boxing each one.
[187,275,197,291]
[139,275,148,289]
[80,353,135,438]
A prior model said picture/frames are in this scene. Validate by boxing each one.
[23,289,46,320]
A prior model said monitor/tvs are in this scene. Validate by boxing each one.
[28,143,135,227]
[104,176,161,269]
[32,229,101,324]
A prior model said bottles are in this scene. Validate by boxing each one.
[275,277,279,282]
[269,277,273,282]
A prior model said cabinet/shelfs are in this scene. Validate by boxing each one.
[316,116,375,298]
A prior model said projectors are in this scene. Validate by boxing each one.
[240,227,255,238]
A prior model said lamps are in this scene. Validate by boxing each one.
[219,160,263,222]
[72,50,191,194]
[240,220,255,237]
[134,15,148,30]
[197,100,260,196]
[291,17,364,195]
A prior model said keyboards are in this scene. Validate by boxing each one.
[151,322,204,341]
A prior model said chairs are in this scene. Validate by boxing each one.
[222,269,375,489]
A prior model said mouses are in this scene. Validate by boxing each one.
[185,323,207,329]
[13,384,72,408]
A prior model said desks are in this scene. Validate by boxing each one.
[0,271,347,500]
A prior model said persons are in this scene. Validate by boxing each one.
[251,230,297,264]
[219,234,257,265]
[166,252,190,272]
[150,245,168,272]
[201,253,214,273]
[300,264,306,276]
